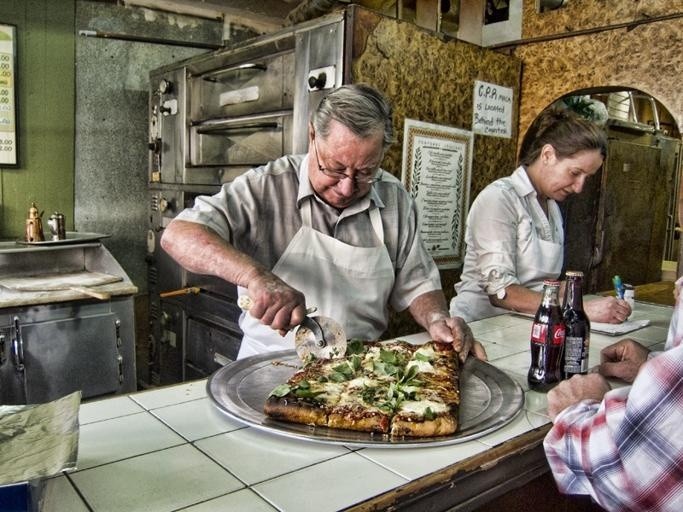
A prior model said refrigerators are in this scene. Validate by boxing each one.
[562,138,663,294]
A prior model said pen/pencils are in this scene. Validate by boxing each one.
[611,274,624,301]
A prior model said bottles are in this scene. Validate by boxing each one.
[528,279,560,391]
[563,270,590,377]
[47,211,66,241]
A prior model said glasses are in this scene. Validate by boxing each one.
[314,140,384,186]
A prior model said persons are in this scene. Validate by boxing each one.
[161,79,488,366]
[449,105,632,325]
[543,174,683,511]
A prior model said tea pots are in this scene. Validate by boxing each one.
[24,202,45,244]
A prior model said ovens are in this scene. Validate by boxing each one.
[144,5,527,390]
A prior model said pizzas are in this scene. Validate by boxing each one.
[263,339,460,439]
